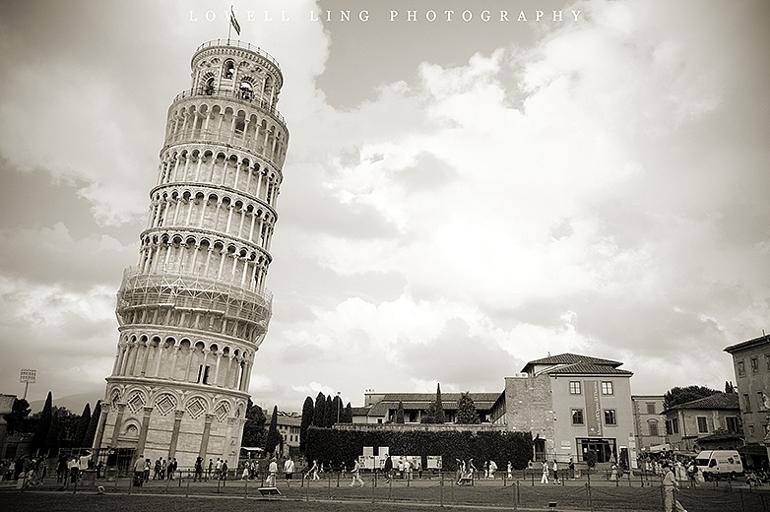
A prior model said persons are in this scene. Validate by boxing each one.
[507,460,513,479]
[551,458,561,484]
[661,463,688,512]
[383,453,392,483]
[395,456,498,486]
[567,457,577,480]
[0,449,365,491]
[608,445,770,489]
[540,459,549,483]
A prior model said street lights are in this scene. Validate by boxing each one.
[18,368,38,402]
[336,390,342,427]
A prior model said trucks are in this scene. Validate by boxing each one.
[686,448,744,480]
